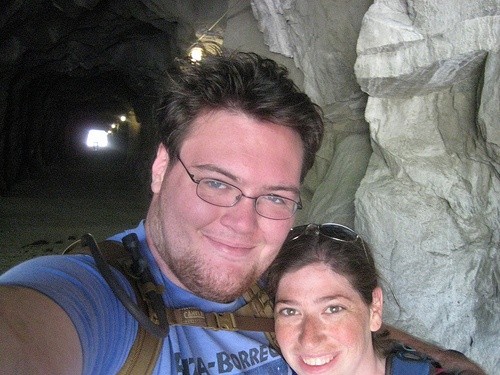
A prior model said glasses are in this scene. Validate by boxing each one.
[175,151,304,221]
[283,222,372,267]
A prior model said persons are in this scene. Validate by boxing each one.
[0,52,485,375]
[268,223,446,375]
